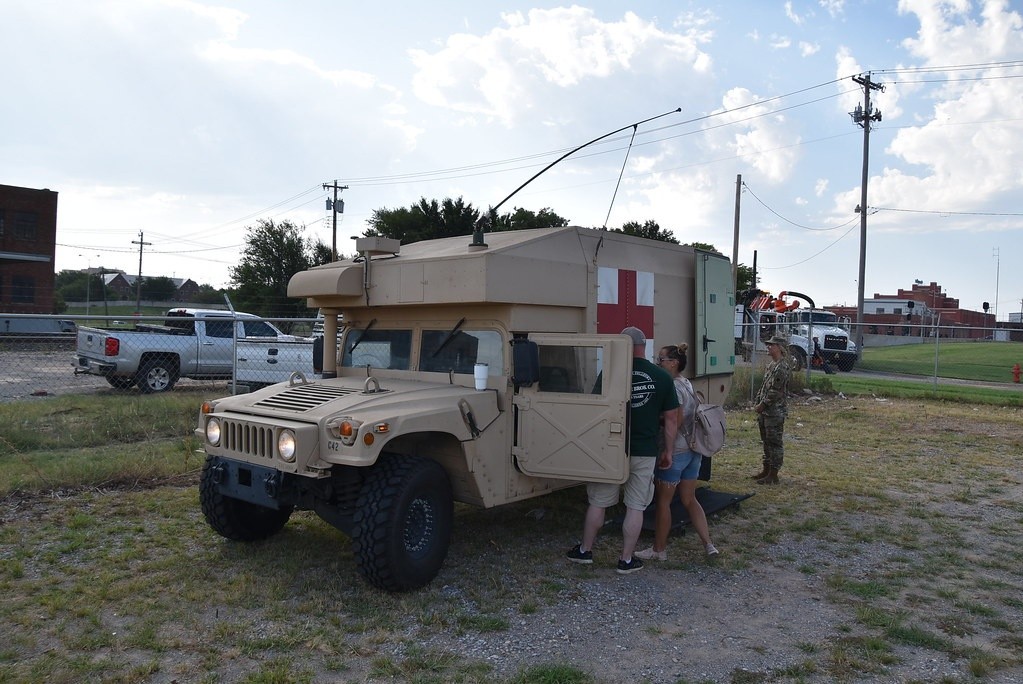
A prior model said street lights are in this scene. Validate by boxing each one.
[79,253,101,323]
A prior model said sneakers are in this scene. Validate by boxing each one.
[634,546,668,562]
[758,468,780,484]
[566,544,593,564]
[751,466,770,479]
[705,543,719,556]
[616,554,644,575]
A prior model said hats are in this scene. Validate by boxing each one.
[622,326,647,345]
[764,335,789,348]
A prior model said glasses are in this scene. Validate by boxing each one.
[657,357,679,364]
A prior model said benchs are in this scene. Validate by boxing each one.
[136,323,191,335]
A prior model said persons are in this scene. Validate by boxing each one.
[566,325,681,573]
[635,341,719,562]
[748,336,793,485]
[813,337,836,374]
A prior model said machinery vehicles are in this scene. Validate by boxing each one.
[735,288,857,374]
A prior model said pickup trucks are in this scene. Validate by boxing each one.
[233,308,393,394]
[69,308,286,394]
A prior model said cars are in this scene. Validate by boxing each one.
[986,335,993,340]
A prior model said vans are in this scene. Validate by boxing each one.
[113,321,126,325]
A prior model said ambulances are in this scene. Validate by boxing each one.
[199,226,740,597]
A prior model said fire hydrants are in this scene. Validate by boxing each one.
[1012,363,1022,382]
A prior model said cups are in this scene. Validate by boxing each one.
[474,363,489,392]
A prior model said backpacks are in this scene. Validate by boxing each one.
[673,378,726,457]
